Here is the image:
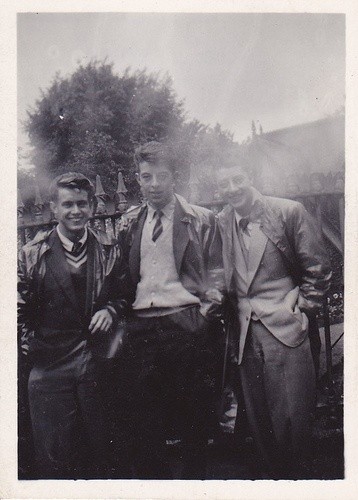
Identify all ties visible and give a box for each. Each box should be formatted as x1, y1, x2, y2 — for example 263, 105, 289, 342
152, 209, 163, 243
241, 218, 248, 238
71, 242, 82, 258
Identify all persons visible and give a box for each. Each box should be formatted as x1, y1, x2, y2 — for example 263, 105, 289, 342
213, 150, 336, 479
17, 170, 124, 480
114, 139, 229, 480
